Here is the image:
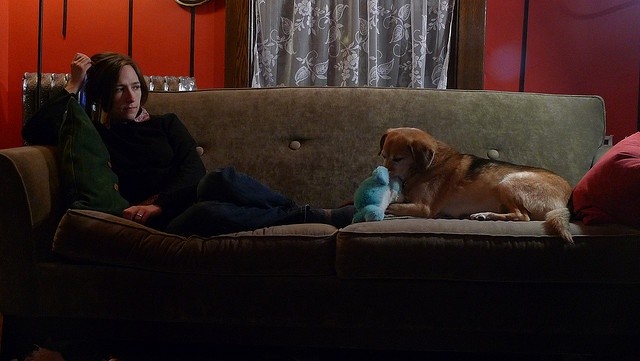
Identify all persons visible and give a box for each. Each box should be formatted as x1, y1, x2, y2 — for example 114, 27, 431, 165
17, 52, 356, 235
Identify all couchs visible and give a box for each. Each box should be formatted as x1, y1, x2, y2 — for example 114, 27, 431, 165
0, 85, 640, 331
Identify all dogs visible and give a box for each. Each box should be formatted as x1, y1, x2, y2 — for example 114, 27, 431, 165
378, 127, 576, 247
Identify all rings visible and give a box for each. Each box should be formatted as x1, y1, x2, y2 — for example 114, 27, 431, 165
136, 214, 143, 218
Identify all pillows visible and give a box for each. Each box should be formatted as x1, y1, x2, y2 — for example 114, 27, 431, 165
570, 128, 640, 228
54, 97, 129, 219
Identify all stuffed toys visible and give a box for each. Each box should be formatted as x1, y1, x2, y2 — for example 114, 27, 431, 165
348, 166, 405, 227
